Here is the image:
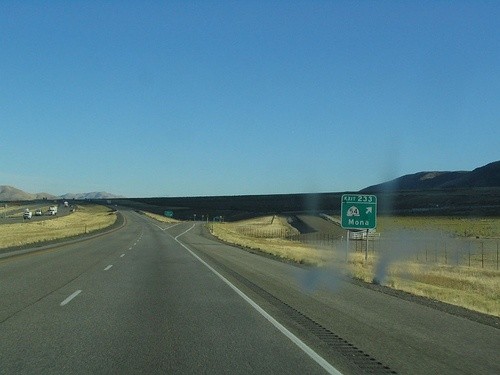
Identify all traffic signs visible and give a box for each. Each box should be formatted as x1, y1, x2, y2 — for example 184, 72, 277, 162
340, 194, 378, 231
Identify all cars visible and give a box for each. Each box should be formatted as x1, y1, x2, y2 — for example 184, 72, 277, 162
347, 228, 380, 242
24, 201, 73, 221
